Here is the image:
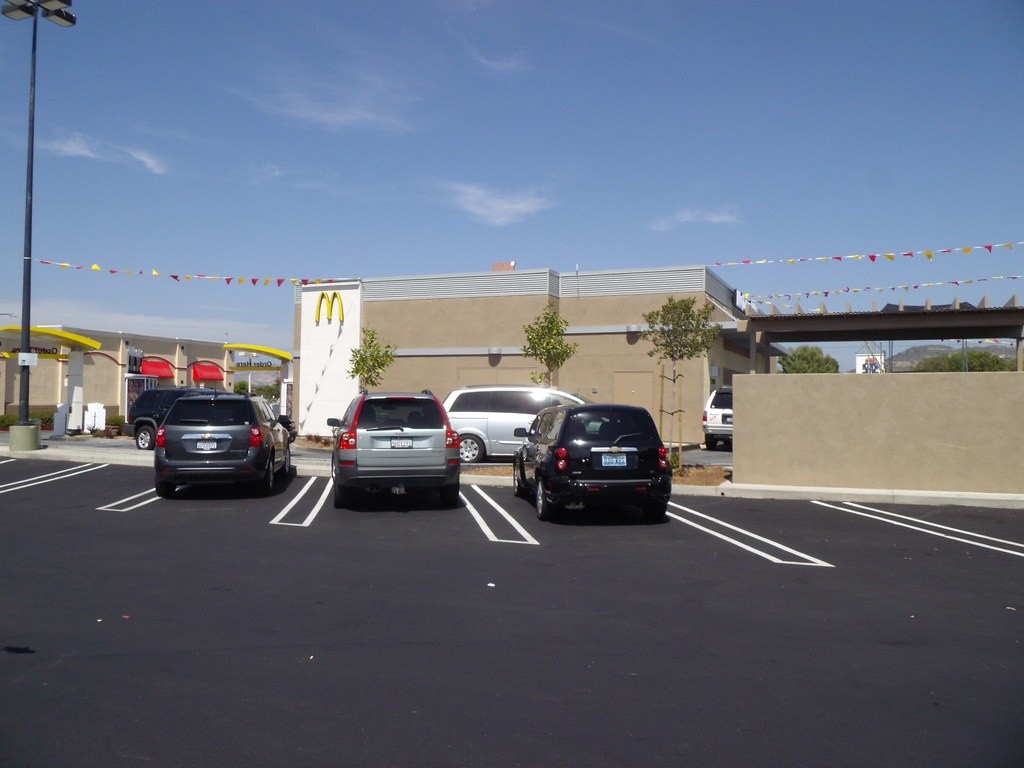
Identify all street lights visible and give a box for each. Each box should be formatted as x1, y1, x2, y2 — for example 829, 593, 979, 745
239, 351, 258, 395
1, 0, 78, 424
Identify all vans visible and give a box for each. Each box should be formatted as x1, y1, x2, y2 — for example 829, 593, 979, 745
444, 386, 610, 463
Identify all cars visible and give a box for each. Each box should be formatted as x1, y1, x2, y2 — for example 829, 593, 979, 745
270, 403, 280, 419
515, 402, 672, 523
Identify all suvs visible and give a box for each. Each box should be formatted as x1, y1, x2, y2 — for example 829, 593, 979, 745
126, 388, 298, 450
153, 395, 292, 496
701, 387, 734, 450
327, 387, 461, 507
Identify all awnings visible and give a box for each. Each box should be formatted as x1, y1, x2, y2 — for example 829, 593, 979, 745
193, 364, 224, 381
141, 361, 174, 379
